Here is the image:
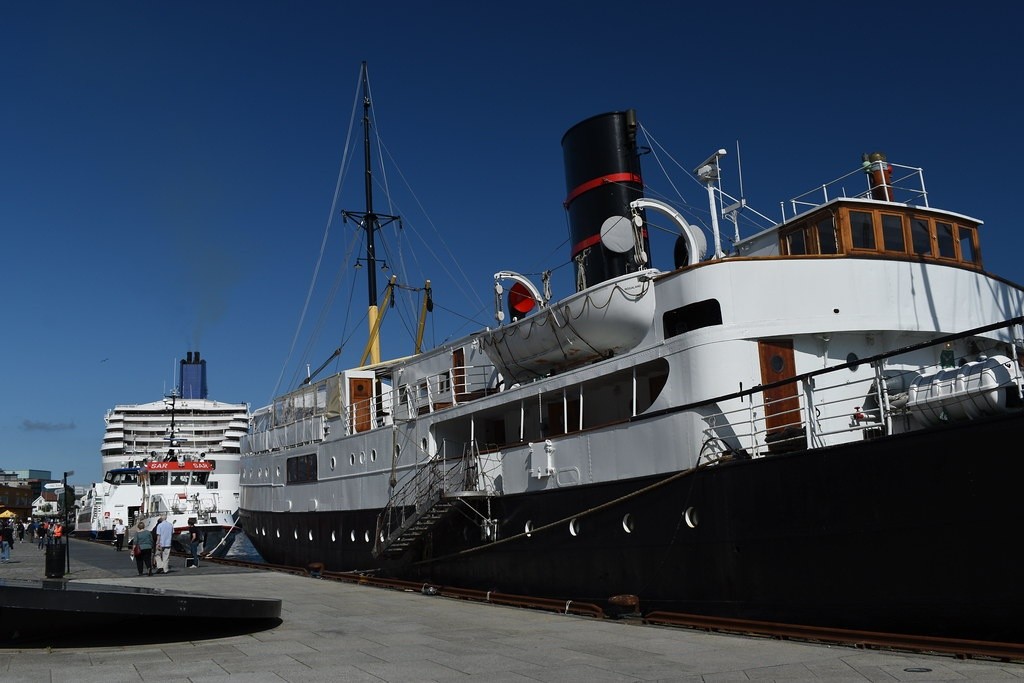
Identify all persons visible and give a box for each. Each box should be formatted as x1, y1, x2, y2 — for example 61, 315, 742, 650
130, 522, 156, 576
0, 519, 63, 564
188, 518, 202, 569
154, 515, 173, 574
114, 519, 126, 551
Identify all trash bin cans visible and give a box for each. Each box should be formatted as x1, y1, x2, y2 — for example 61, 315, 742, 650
45, 542, 67, 578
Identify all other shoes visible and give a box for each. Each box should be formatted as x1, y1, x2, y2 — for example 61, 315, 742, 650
147, 568, 153, 576
155, 568, 163, 574
137, 573, 143, 576
189, 565, 197, 568
163, 572, 167, 574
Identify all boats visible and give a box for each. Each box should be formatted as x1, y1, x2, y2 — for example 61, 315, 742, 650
68, 358, 252, 558
230, 62, 1023, 643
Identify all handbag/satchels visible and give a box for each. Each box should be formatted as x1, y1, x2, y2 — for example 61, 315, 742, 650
134, 544, 141, 555
